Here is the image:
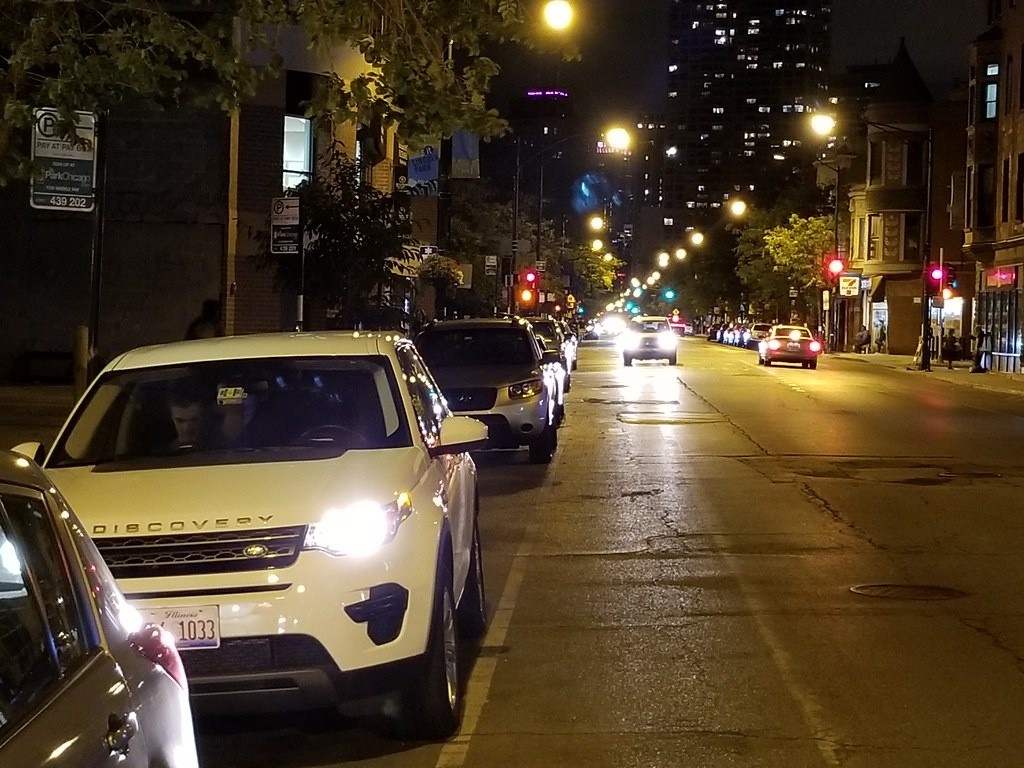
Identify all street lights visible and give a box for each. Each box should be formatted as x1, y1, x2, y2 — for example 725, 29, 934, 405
813, 115, 934, 371
511, 128, 629, 316
773, 148, 840, 354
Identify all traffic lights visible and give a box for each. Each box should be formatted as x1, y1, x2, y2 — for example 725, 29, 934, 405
931, 270, 942, 279
526, 273, 535, 282
521, 289, 532, 301
665, 292, 673, 299
947, 266, 957, 288
829, 260, 843, 273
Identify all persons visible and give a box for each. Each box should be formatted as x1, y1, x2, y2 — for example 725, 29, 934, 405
974, 324, 986, 367
115, 385, 243, 463
856, 325, 871, 353
946, 328, 959, 347
875, 319, 887, 354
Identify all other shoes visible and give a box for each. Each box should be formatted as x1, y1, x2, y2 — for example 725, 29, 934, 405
948, 367, 954, 370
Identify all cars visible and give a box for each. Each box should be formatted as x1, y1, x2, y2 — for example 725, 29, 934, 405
622, 316, 678, 366
758, 325, 820, 369
415, 315, 602, 466
669, 318, 685, 336
707, 323, 773, 349
685, 323, 694, 335
0, 448, 199, 767
11, 331, 488, 734
